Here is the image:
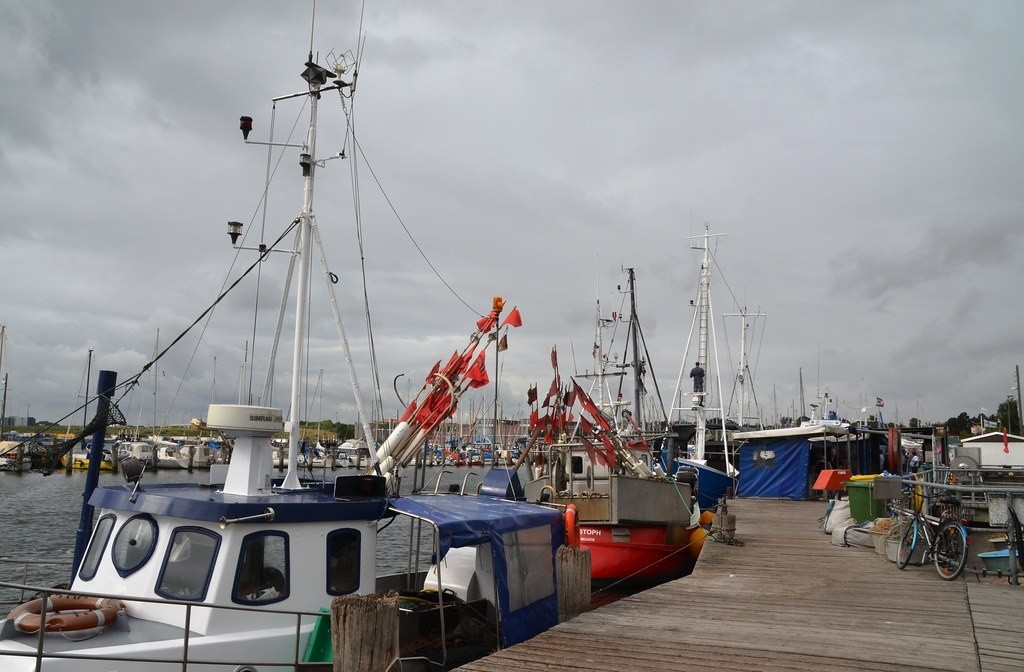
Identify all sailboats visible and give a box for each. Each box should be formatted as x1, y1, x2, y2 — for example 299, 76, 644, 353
0, 0, 567, 672
647, 225, 741, 516
1, 264, 918, 515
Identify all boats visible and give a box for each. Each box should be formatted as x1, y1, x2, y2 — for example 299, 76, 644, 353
471, 346, 713, 585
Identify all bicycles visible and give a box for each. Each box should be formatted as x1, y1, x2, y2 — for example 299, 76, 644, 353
894, 483, 971, 581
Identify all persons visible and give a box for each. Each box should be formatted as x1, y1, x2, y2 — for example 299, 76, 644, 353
901, 448, 920, 474
827, 446, 842, 501
689, 362, 706, 408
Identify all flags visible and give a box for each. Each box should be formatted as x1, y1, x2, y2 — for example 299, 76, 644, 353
876, 398, 883, 406
984, 420, 997, 428
971, 421, 986, 434
499, 334, 507, 353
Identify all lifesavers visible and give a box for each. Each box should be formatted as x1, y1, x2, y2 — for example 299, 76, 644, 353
8, 595, 126, 632
565, 503, 581, 549
